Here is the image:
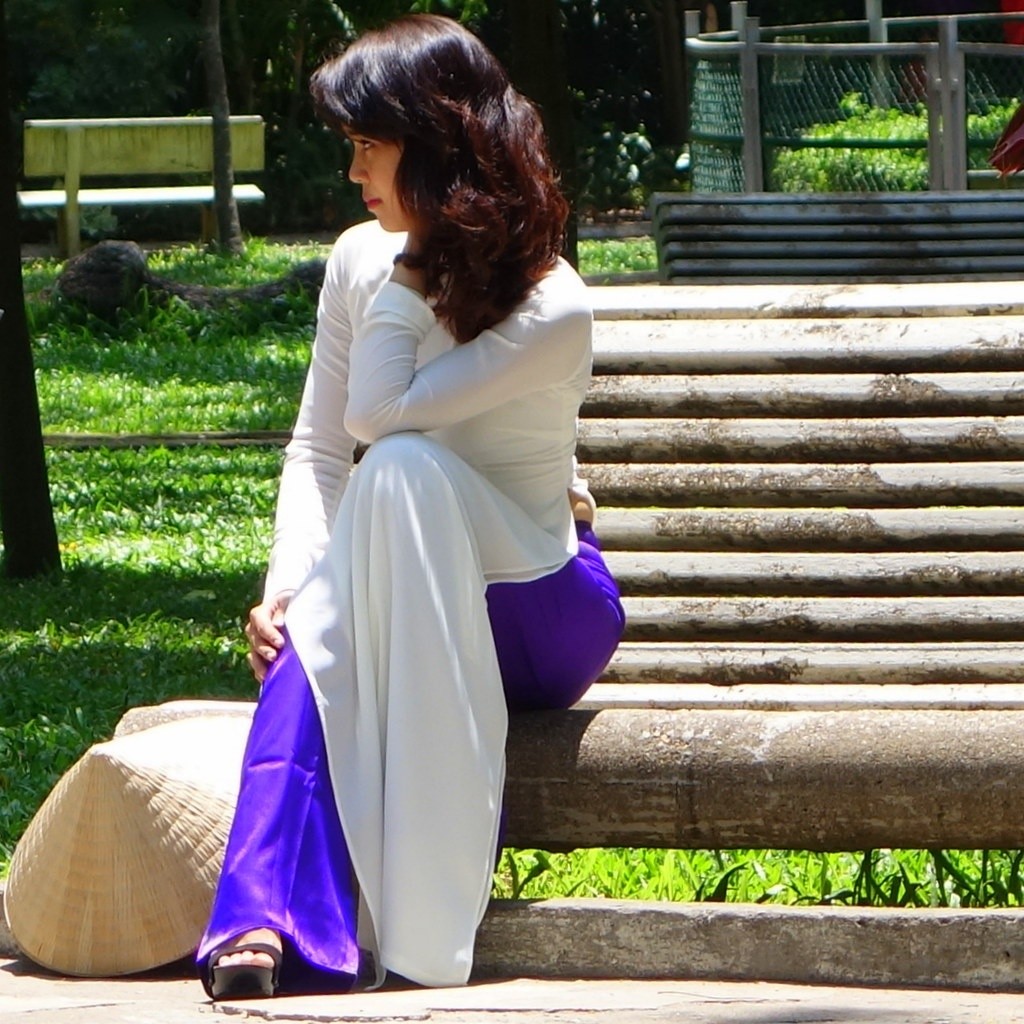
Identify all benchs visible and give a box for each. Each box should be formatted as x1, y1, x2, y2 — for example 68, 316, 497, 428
16, 115, 266, 254
648, 190, 1024, 288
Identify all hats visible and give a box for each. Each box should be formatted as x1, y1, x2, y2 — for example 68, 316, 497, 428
2, 716, 254, 977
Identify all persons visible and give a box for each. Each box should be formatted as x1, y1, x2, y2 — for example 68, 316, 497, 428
196, 13, 626, 1001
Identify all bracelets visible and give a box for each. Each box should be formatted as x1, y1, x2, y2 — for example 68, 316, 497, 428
393, 254, 430, 275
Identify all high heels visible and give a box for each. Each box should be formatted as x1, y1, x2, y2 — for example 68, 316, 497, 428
209, 929, 284, 996
